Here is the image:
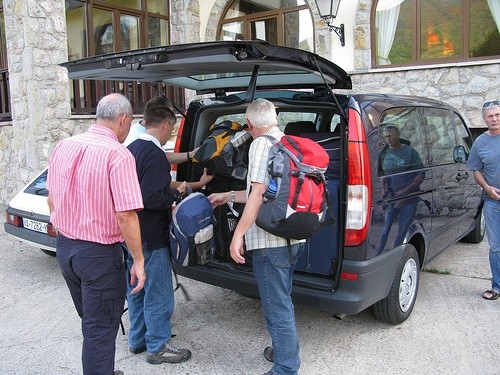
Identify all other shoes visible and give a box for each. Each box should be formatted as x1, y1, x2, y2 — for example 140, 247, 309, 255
262, 371, 274, 375
114, 370, 124, 375
264, 347, 275, 362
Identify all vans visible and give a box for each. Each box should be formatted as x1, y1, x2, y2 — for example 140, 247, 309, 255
59, 37, 486, 327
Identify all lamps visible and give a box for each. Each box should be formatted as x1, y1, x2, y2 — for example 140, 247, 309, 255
306, 0, 344, 45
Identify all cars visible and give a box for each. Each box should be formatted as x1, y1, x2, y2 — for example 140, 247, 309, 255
3, 140, 175, 258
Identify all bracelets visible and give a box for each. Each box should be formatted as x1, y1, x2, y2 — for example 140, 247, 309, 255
230, 191, 235, 202
186, 152, 190, 160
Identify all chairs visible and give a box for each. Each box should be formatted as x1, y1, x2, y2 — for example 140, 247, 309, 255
283, 121, 317, 137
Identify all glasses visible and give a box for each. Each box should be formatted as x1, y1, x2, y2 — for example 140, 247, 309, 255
483, 101, 500, 107
113, 242, 128, 273
383, 126, 394, 130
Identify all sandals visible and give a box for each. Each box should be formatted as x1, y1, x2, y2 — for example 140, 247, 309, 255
483, 290, 500, 300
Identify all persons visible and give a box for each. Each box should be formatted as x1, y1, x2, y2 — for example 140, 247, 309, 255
373, 124, 424, 259
202, 99, 307, 375
468, 101, 500, 300
120, 98, 214, 364
46, 93, 146, 375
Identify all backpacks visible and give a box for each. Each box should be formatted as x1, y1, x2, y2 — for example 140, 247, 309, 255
192, 120, 254, 192
168, 185, 215, 267
248, 135, 330, 240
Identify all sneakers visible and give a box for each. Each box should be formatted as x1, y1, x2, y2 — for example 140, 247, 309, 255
147, 341, 192, 364
130, 345, 147, 354
